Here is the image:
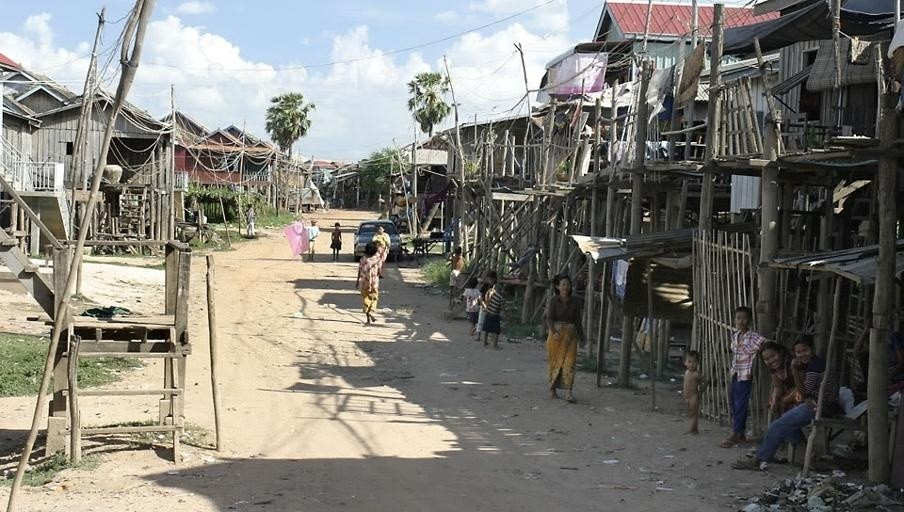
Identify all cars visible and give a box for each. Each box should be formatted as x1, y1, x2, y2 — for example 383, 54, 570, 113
354, 219, 403, 262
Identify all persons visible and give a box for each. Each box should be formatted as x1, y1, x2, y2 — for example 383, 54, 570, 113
355, 243, 382, 321
329, 222, 342, 260
307, 219, 319, 259
737, 334, 840, 470
446, 247, 464, 306
244, 204, 256, 236
720, 307, 766, 448
461, 271, 503, 350
371, 225, 391, 278
682, 351, 709, 435
546, 274, 587, 402
759, 342, 796, 427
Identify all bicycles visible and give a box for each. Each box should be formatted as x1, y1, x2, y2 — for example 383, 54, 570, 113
396, 238, 427, 268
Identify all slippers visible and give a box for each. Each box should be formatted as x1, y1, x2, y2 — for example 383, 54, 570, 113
565, 395, 577, 403
719, 439, 742, 448
